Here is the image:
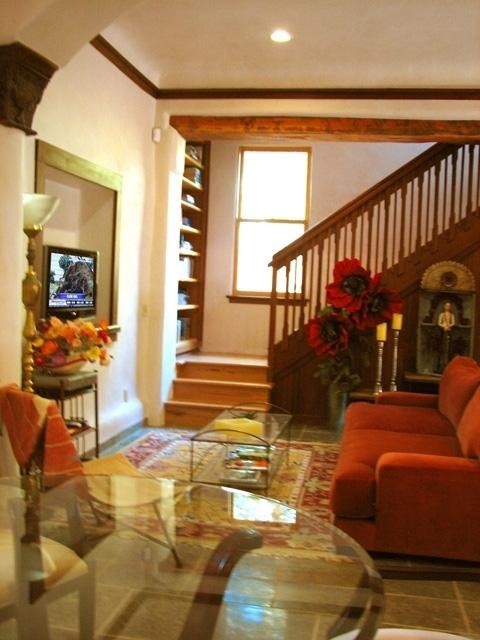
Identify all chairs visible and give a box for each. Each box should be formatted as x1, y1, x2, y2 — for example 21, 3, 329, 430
0, 380, 184, 570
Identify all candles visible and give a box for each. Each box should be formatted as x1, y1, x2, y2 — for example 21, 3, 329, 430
390, 312, 405, 331
375, 322, 388, 343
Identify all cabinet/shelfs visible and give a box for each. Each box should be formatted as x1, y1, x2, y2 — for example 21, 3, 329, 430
21, 367, 101, 464
175, 136, 211, 357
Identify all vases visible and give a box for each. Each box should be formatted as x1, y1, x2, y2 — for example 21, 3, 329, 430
36, 354, 90, 379
325, 378, 354, 433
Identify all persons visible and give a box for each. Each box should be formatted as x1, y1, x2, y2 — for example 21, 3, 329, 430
55, 255, 93, 297
433, 302, 456, 374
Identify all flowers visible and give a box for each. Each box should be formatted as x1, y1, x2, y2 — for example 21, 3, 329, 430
299, 254, 408, 391
29, 308, 115, 375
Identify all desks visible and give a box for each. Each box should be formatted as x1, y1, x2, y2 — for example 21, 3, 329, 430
347, 386, 402, 406
399, 369, 442, 394
0, 470, 388, 638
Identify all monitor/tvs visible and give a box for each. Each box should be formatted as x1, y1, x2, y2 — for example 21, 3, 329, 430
42, 245, 99, 317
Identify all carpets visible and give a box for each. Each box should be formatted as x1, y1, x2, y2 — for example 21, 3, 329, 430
106, 429, 344, 562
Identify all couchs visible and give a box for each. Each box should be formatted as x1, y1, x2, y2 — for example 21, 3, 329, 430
324, 352, 479, 579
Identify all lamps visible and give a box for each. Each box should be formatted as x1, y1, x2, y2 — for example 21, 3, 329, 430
18, 190, 59, 392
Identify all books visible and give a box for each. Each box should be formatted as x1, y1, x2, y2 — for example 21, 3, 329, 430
176, 142, 203, 343
220, 445, 277, 484
